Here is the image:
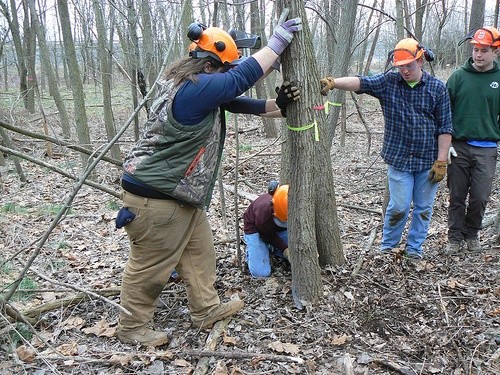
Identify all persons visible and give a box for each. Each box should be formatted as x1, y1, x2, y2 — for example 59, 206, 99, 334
320, 38, 453, 258
446, 26, 500, 254
117, 7, 303, 347
243, 184, 290, 278
168, 81, 300, 283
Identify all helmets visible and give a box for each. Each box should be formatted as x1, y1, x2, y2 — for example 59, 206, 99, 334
273, 185, 288, 222
392, 38, 424, 67
470, 26, 500, 46
188, 27, 239, 66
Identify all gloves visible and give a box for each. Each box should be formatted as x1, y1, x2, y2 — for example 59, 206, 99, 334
272, 56, 282, 72
267, 8, 302, 55
429, 160, 447, 184
275, 81, 300, 108
320, 77, 335, 96
447, 143, 458, 165
280, 107, 287, 118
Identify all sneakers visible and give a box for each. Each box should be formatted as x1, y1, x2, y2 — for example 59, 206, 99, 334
466, 239, 481, 253
118, 327, 171, 347
404, 253, 424, 271
194, 300, 244, 328
445, 240, 464, 255
